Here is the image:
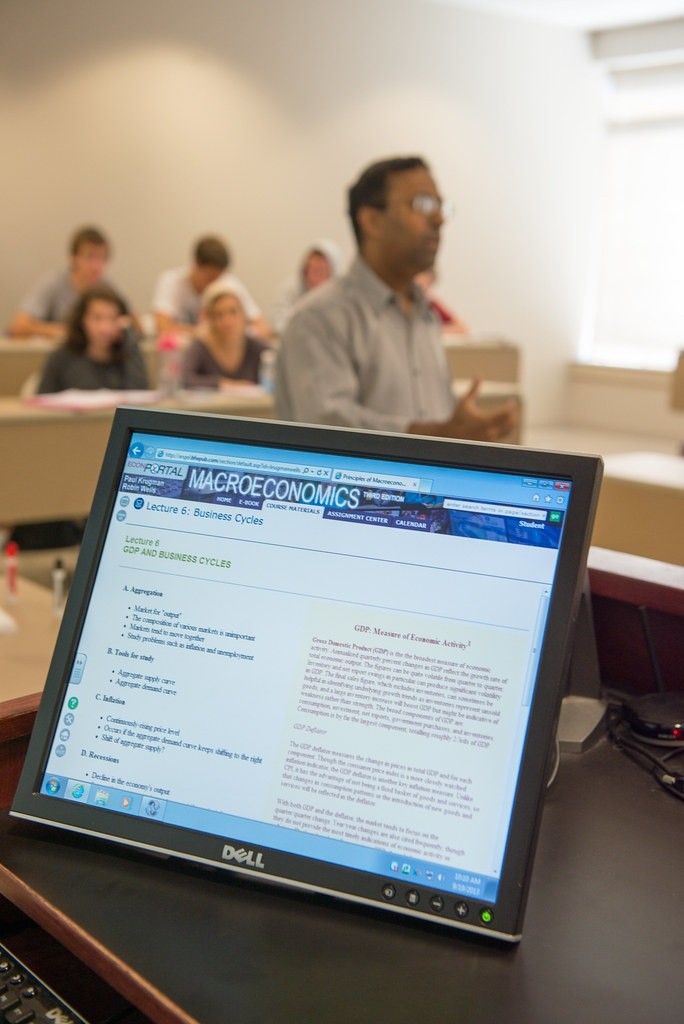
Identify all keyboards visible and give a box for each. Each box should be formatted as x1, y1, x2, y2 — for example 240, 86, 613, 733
0, 945, 88, 1024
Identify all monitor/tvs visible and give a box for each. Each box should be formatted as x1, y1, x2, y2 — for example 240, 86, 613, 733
7, 407, 604, 953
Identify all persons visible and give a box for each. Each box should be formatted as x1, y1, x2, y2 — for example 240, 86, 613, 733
413, 268, 462, 334
9, 226, 143, 339
37, 283, 147, 393
272, 158, 518, 442
144, 239, 271, 349
181, 277, 275, 393
300, 249, 332, 291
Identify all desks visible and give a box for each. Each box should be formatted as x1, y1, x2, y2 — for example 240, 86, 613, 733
0, 324, 683, 709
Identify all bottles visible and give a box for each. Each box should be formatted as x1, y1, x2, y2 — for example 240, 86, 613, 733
3, 541, 23, 604
155, 339, 183, 398
50, 556, 69, 617
258, 351, 277, 399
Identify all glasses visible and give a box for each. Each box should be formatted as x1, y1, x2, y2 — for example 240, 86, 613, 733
410, 196, 443, 216
